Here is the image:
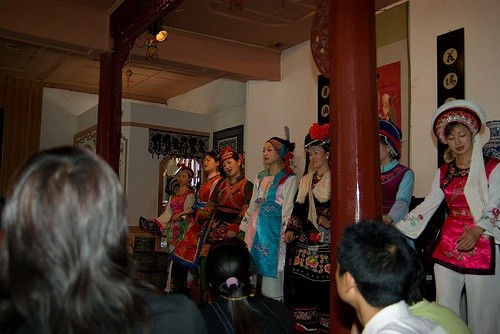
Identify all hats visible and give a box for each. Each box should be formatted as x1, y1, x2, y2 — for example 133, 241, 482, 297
378, 118, 403, 159
430, 100, 486, 145
174, 163, 194, 178
203, 148, 221, 162
219, 148, 246, 168
304, 123, 331, 152
266, 136, 295, 185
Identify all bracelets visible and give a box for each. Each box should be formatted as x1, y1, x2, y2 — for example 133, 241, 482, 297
184, 210, 187, 214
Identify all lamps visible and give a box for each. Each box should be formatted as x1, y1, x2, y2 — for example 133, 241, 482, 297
147, 17, 167, 42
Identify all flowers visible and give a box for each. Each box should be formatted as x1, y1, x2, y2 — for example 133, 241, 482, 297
308, 122, 330, 142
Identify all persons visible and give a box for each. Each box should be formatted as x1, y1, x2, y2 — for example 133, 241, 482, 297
238, 125, 298, 303
150, 146, 254, 292
0, 146, 208, 334
202, 238, 295, 334
395, 98, 500, 334
284, 122, 332, 334
336, 217, 472, 334
378, 119, 414, 253
